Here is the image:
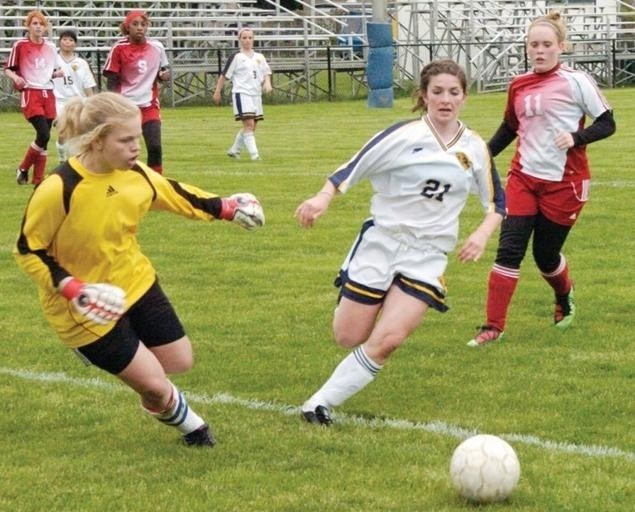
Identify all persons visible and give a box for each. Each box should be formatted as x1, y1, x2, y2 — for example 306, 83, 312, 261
294, 60, 509, 429
11, 92, 266, 449
3, 10, 65, 186
102, 11, 170, 176
53, 30, 97, 166
466, 11, 617, 348
212, 28, 273, 162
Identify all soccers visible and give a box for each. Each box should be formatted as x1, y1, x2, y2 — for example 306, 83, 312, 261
449, 434, 521, 503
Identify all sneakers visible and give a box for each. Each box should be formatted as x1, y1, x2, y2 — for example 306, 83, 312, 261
299, 406, 333, 425
182, 424, 214, 448
467, 324, 504, 347
251, 154, 262, 160
553, 281, 576, 330
228, 148, 241, 159
16, 167, 28, 185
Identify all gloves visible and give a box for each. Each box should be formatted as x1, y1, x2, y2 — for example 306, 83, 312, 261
219, 193, 266, 232
61, 277, 125, 325
